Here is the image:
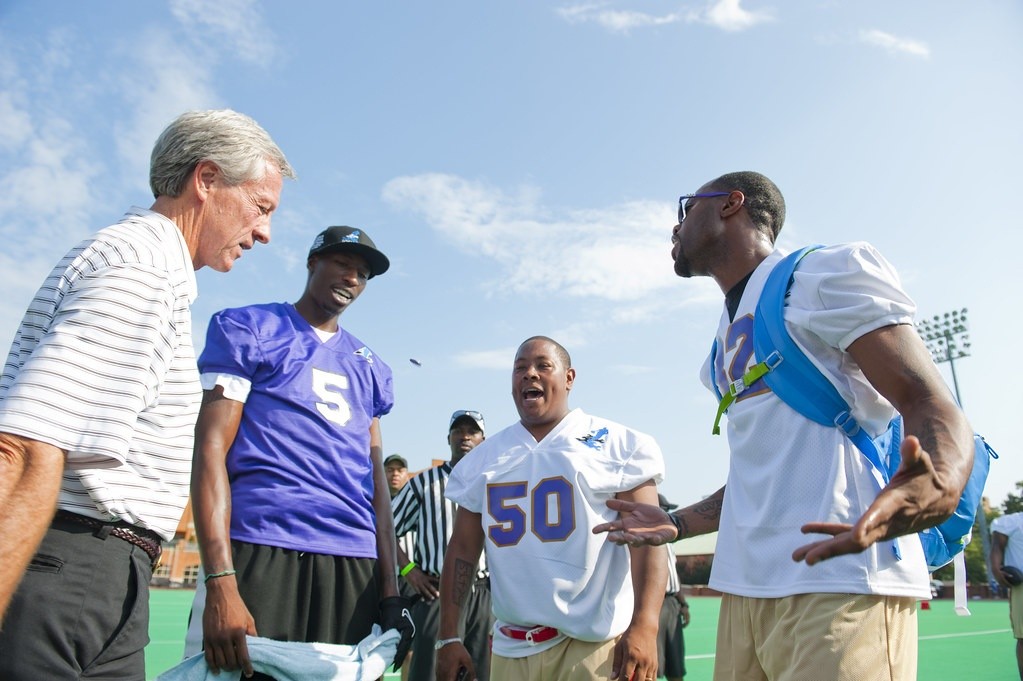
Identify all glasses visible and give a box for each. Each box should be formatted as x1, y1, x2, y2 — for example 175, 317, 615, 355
677, 193, 746, 226
451, 413, 484, 425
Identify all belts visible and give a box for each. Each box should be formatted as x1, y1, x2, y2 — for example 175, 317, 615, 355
54, 508, 161, 560
499, 623, 564, 646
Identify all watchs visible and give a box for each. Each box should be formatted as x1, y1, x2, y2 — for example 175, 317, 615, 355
435, 638, 461, 650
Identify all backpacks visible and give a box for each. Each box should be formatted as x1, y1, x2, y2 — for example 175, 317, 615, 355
711, 246, 998, 574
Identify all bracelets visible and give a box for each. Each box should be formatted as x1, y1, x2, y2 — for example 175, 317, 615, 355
401, 563, 416, 577
205, 570, 236, 583
681, 602, 688, 607
667, 512, 682, 542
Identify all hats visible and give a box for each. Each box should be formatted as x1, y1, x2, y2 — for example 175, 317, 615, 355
384, 455, 407, 468
448, 410, 485, 438
307, 226, 390, 279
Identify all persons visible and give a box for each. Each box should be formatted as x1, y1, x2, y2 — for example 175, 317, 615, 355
389, 410, 492, 681
0, 110, 295, 681
384, 455, 417, 681
990, 510, 1023, 681
658, 494, 690, 681
434, 336, 670, 681
183, 227, 415, 681
593, 170, 975, 681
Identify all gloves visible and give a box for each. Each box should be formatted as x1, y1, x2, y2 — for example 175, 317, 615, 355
377, 596, 416, 674
